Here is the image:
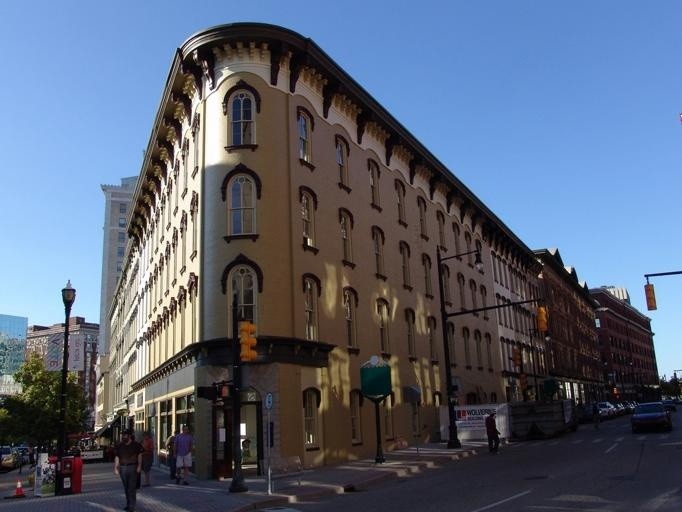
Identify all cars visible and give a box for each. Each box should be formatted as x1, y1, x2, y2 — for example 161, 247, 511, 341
597, 400, 639, 420
662, 399, 677, 413
630, 403, 673, 434
0, 446, 43, 472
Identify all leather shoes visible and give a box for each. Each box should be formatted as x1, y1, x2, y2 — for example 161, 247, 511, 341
123, 505, 136, 511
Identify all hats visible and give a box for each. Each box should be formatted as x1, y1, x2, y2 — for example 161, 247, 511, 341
120, 429, 135, 436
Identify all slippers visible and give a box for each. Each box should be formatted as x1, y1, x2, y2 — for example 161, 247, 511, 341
183, 480, 189, 485
175, 478, 181, 483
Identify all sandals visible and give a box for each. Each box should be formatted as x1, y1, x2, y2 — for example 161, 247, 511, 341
141, 483, 150, 486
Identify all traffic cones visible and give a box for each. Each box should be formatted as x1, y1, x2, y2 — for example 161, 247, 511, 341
14, 480, 24, 497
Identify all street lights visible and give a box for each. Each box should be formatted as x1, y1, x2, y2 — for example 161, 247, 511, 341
435, 240, 484, 450
54, 279, 76, 498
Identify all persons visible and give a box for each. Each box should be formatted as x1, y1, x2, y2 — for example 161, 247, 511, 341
173, 425, 194, 486
165, 430, 181, 481
140, 431, 154, 488
592, 401, 602, 431
484, 411, 503, 456
113, 428, 145, 512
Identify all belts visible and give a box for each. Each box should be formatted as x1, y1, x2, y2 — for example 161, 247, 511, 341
120, 462, 137, 466
